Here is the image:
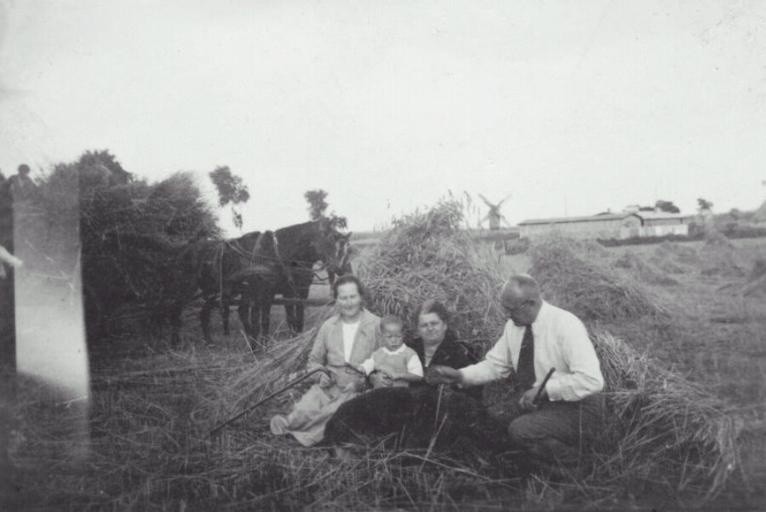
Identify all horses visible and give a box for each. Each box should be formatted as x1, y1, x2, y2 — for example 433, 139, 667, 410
168, 214, 356, 352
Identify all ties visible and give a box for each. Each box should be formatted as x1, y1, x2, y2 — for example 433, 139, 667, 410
517, 325, 536, 388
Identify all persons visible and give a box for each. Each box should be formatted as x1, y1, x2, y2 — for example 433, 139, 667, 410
270, 277, 382, 447
410, 300, 483, 401
425, 273, 604, 478
357, 317, 424, 389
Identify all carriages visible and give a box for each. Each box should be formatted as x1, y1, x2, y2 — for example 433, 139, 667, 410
0, 183, 353, 349
